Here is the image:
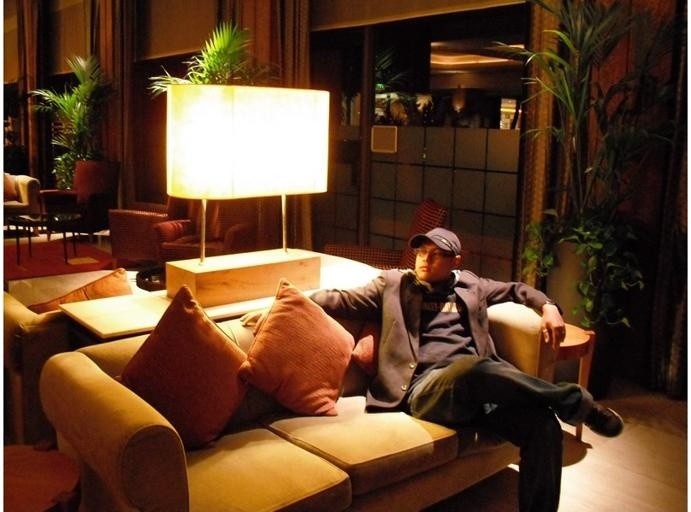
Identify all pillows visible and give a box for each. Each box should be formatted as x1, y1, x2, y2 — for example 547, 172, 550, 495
351, 315, 380, 378
117, 284, 255, 449
26, 264, 133, 315
234, 275, 357, 418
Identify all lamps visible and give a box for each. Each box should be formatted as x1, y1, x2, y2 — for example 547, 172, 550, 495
161, 82, 336, 310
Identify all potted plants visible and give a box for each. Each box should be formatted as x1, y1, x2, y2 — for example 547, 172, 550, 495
483, 0, 686, 400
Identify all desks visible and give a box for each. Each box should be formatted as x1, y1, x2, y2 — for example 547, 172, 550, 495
5, 439, 87, 511
554, 321, 598, 445
54, 264, 380, 353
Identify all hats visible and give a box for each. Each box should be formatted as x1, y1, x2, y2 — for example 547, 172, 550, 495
408, 226, 462, 258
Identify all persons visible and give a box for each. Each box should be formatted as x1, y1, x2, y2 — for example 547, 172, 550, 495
240, 228, 625, 512
4, 131, 26, 174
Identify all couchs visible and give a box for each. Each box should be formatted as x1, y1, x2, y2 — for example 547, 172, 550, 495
38, 273, 563, 511
3, 266, 139, 444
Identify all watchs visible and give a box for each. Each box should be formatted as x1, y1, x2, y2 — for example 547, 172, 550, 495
546, 299, 556, 305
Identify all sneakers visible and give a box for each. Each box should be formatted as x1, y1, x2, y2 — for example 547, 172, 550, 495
585, 403, 624, 438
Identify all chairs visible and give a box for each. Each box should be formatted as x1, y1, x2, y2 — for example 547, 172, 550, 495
321, 195, 462, 277
3, 151, 290, 270
